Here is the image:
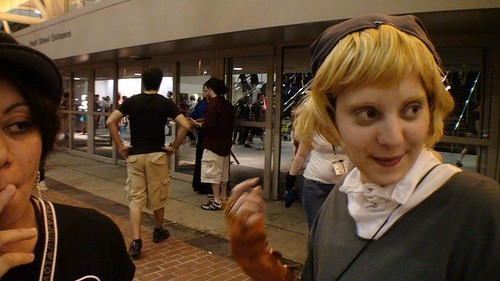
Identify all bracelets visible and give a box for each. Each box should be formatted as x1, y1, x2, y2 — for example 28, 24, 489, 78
283, 264, 300, 281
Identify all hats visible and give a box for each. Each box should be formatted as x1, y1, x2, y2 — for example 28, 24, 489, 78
0, 30, 63, 110
309, 12, 441, 74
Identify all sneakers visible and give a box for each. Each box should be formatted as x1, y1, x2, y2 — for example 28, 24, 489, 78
126, 240, 140, 256
153, 229, 170, 242
201, 201, 221, 210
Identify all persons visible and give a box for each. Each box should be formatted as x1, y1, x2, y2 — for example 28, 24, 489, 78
36, 76, 354, 234
456, 102, 482, 167
222, 13, 500, 281
107, 64, 193, 259
0, 30, 138, 281
